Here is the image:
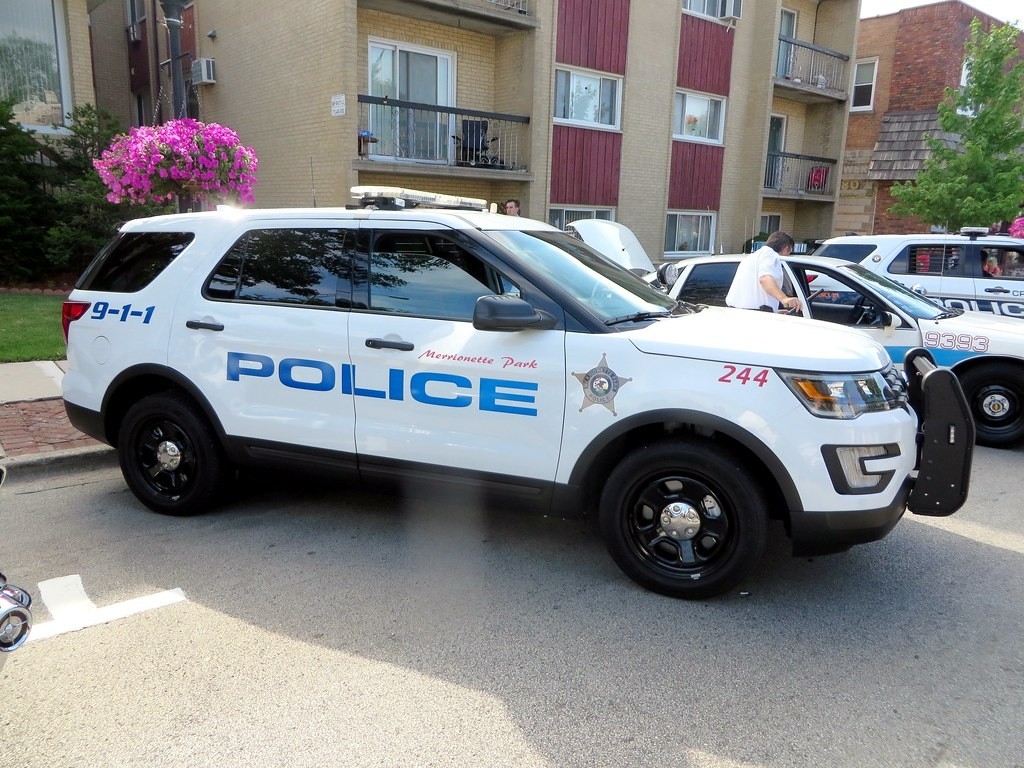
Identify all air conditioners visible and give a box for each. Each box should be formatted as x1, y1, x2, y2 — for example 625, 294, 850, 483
719, 0, 743, 20
191, 58, 213, 82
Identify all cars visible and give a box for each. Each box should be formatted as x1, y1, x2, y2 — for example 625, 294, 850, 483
61, 185, 919, 599
565, 219, 1024, 449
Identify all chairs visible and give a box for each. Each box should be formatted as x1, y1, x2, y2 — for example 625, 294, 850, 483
452, 120, 498, 162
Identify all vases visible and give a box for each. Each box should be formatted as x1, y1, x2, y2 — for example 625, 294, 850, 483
178, 178, 202, 192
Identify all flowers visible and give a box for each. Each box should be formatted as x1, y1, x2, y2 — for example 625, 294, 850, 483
92, 118, 258, 211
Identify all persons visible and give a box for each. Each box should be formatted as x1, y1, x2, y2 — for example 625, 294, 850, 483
725, 231, 803, 313
505, 199, 521, 217
982, 250, 1000, 278
